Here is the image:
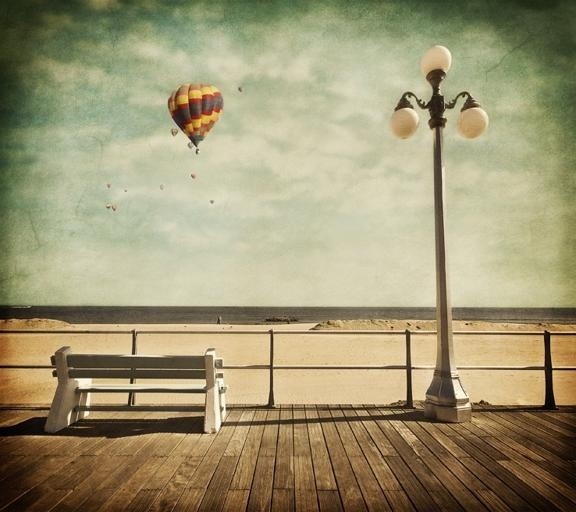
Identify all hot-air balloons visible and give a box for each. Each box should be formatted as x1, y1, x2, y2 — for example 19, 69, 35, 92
96, 82, 242, 215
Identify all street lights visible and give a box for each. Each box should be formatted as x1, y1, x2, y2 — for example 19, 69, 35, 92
384, 45, 493, 425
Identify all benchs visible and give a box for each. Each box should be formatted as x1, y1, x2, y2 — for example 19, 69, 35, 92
44, 346, 228, 434
